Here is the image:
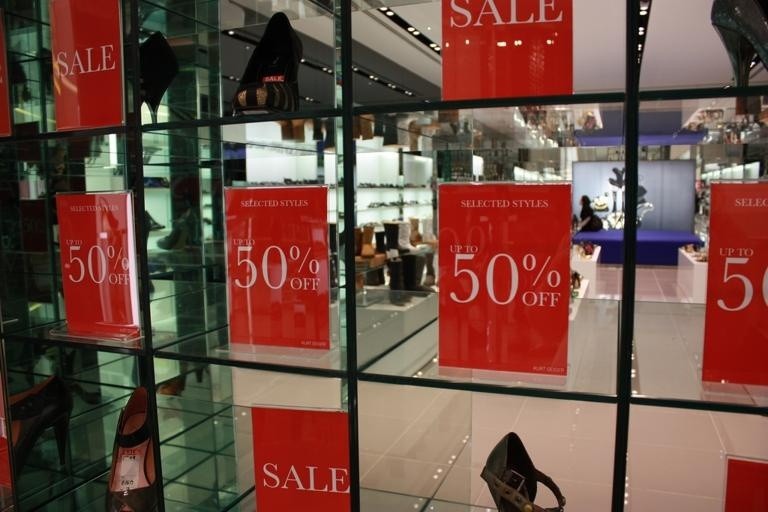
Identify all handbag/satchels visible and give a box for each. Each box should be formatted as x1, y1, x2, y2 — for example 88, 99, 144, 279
588, 215, 602, 231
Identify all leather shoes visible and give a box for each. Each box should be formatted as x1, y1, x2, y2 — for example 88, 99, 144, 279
480, 432, 566, 512
73, 384, 107, 406
105, 386, 160, 512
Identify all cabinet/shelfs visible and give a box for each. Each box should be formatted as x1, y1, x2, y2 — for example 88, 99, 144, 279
1, 1, 768, 512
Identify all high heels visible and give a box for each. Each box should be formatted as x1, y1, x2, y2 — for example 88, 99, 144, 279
157, 219, 194, 251
139, 30, 179, 124
155, 366, 204, 396
231, 12, 303, 117
710, 0, 768, 115
9, 374, 73, 481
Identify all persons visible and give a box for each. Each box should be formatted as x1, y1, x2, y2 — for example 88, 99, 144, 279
577, 194, 595, 260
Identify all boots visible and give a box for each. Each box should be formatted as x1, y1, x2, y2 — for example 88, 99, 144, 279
352, 113, 409, 145
276, 117, 335, 149
353, 219, 436, 306
